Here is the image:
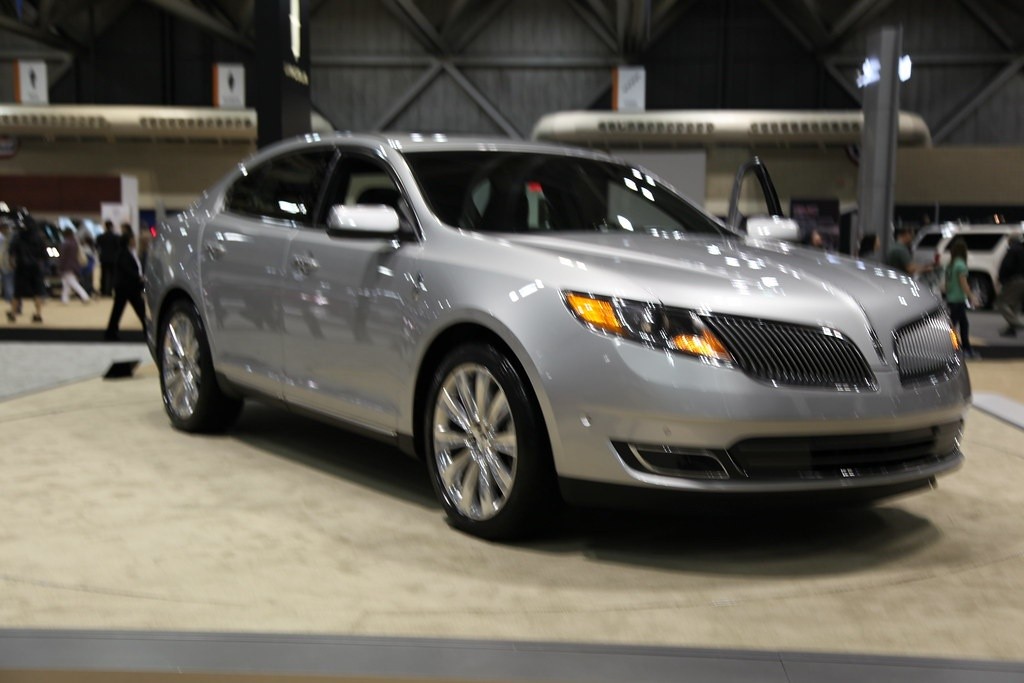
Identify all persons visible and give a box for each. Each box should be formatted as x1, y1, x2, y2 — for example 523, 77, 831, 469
945, 239, 981, 357
858, 227, 940, 296
207, 249, 374, 343
998, 233, 1024, 337
0, 218, 147, 334
792, 198, 823, 245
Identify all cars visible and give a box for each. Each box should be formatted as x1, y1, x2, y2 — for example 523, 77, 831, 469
137, 130, 977, 546
910, 221, 1024, 311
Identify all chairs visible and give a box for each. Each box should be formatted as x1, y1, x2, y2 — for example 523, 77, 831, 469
539, 168, 596, 232
355, 186, 403, 217
431, 171, 487, 231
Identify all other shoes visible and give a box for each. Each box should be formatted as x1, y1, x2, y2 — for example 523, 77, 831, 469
6, 311, 14, 321
33, 315, 42, 321
